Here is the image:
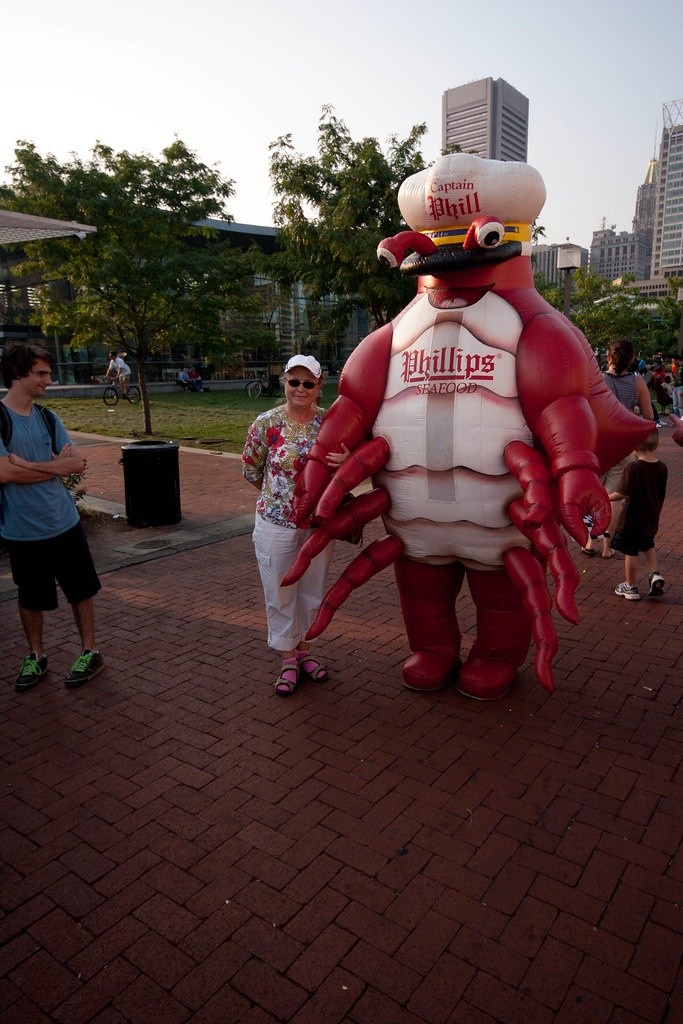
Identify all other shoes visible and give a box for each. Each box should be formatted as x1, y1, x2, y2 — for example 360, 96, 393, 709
122, 393, 128, 399
656, 423, 661, 427
659, 419, 668, 426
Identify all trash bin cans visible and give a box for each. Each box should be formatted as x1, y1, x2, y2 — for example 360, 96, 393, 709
121, 441, 182, 528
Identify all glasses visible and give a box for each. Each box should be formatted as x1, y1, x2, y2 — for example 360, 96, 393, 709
285, 378, 320, 389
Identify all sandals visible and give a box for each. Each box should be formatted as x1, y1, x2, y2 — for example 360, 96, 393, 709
295, 654, 328, 683
274, 665, 300, 696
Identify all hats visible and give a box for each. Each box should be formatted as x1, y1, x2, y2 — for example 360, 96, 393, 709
284, 354, 322, 379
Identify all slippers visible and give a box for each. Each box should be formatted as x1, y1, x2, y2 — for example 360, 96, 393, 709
602, 549, 615, 559
580, 546, 595, 554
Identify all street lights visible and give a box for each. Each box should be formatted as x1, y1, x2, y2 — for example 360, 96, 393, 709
554, 245, 583, 321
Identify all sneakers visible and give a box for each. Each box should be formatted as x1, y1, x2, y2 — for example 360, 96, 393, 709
615, 582, 641, 600
15, 651, 49, 689
64, 648, 106, 687
648, 571, 665, 597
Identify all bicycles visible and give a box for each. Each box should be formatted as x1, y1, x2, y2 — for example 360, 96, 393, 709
245, 370, 281, 399
102, 377, 141, 407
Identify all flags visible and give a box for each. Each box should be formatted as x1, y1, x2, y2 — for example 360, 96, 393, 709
571, 514, 611, 541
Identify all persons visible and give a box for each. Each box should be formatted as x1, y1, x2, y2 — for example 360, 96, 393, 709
242, 354, 351, 697
107, 351, 131, 395
582, 339, 654, 557
629, 351, 683, 448
0, 341, 106, 691
609, 429, 668, 599
189, 366, 203, 392
177, 367, 192, 392
